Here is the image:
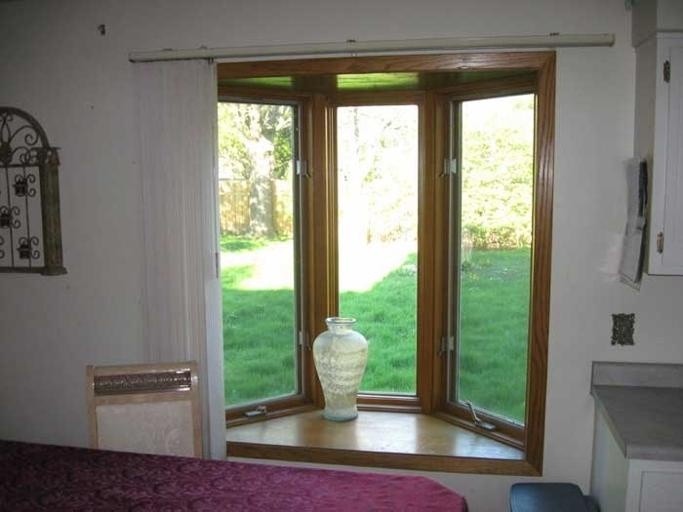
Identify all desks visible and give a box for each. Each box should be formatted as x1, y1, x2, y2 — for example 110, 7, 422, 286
1, 440, 469, 512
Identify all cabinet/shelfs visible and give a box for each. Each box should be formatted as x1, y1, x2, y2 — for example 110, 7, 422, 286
589, 402, 683, 512
630, 0, 683, 276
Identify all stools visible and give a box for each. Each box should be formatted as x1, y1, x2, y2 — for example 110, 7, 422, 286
509, 482, 590, 512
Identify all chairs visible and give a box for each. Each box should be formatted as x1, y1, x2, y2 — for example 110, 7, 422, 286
85, 361, 203, 459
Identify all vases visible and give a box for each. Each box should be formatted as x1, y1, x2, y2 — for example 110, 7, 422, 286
312, 317, 368, 422
